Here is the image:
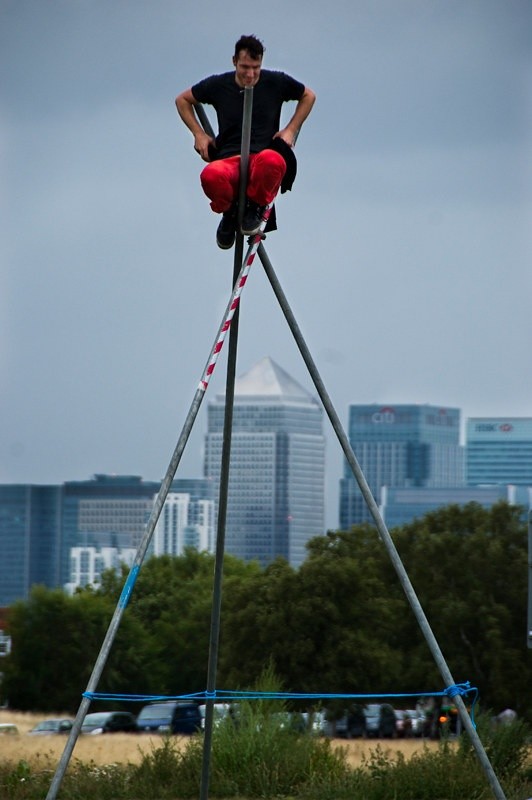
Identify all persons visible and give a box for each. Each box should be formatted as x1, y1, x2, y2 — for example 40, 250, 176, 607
174, 35, 316, 249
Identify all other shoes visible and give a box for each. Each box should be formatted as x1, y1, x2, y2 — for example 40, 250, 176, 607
216, 215, 235, 248
241, 201, 265, 235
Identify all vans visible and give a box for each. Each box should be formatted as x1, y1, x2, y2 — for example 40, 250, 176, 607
134, 701, 241, 736
79, 712, 139, 735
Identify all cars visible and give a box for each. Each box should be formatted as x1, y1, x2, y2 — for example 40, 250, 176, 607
27, 719, 72, 734
268, 702, 466, 738
